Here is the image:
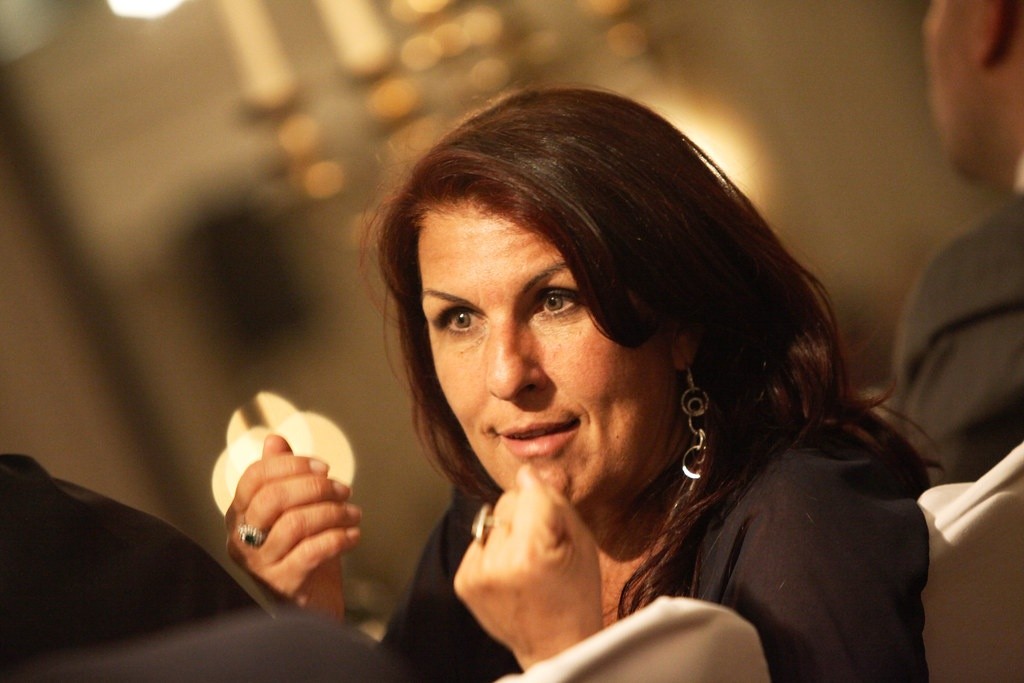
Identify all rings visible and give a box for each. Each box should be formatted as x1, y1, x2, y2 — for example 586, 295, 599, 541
238, 513, 265, 547
471, 500, 507, 545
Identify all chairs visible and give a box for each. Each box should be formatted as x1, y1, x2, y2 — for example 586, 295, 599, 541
495, 597, 773, 683
914, 435, 1023, 682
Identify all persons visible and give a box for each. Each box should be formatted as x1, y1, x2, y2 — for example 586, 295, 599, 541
0, 447, 268, 683
226, 86, 930, 683
895, 0, 1024, 488
